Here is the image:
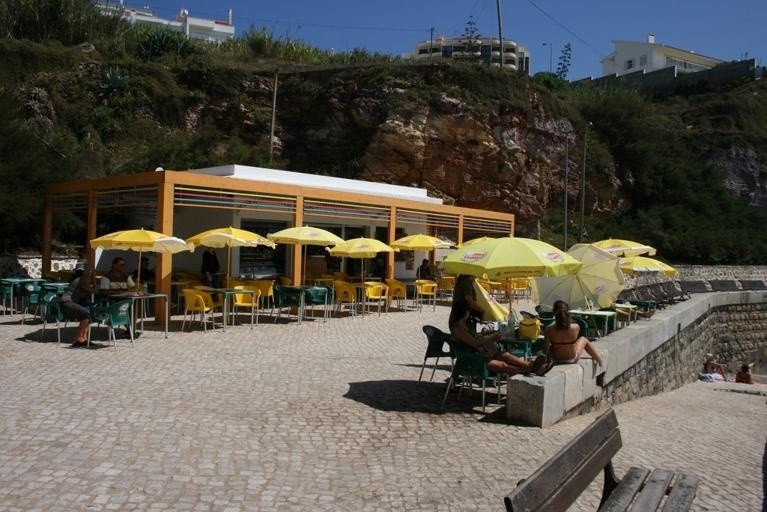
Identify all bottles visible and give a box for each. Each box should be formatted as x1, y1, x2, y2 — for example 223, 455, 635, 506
488, 311, 515, 337
135, 283, 148, 292
587, 300, 594, 312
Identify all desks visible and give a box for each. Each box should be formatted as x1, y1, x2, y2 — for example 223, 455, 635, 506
541, 299, 660, 336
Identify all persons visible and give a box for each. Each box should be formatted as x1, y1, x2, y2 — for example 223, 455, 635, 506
704, 354, 727, 379
446, 296, 554, 378
100, 256, 151, 339
735, 360, 756, 384
443, 274, 485, 386
200, 245, 219, 314
58, 261, 97, 346
543, 300, 604, 367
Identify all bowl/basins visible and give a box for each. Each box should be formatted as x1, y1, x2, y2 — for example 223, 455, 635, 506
240, 273, 254, 279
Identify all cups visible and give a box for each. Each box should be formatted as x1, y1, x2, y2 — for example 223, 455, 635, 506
625, 301, 630, 305
128, 291, 142, 295
110, 282, 127, 287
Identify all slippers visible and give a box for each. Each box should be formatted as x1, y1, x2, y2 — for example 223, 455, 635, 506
532, 353, 554, 376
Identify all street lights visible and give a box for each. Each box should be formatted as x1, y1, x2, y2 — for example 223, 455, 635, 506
556, 122, 572, 252
541, 42, 552, 73
266, 66, 280, 166
579, 119, 592, 244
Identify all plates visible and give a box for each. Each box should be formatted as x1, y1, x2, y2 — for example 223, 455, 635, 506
110, 287, 127, 289
129, 295, 144, 297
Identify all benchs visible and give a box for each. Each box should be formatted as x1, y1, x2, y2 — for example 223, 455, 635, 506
502, 407, 701, 512
619, 279, 767, 312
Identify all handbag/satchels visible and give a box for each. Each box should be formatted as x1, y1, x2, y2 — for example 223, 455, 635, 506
517, 317, 541, 340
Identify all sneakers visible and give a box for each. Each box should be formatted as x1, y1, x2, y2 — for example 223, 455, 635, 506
70, 339, 97, 348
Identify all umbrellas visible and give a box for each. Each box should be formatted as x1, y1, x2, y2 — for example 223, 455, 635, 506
441, 231, 582, 315
612, 253, 679, 297
589, 237, 659, 257
528, 241, 627, 337
